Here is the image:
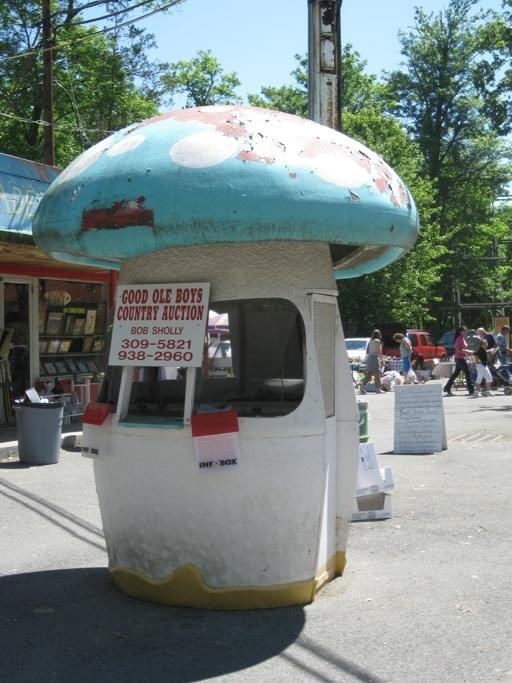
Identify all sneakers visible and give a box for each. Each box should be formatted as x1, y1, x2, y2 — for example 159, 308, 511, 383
444, 386, 497, 398
359, 386, 384, 394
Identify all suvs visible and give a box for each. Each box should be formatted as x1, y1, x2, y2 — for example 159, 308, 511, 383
379, 321, 447, 361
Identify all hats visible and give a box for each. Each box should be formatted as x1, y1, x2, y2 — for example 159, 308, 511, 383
477, 327, 486, 334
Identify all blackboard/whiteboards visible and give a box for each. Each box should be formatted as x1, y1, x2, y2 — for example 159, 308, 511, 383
394, 384, 442, 453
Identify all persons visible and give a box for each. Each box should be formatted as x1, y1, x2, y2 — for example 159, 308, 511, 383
358, 328, 387, 394
393, 332, 412, 383
444, 324, 511, 397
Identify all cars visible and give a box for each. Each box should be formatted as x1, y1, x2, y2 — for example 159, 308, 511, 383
345, 336, 371, 368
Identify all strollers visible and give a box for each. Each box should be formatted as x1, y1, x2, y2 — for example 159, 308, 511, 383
467, 344, 512, 395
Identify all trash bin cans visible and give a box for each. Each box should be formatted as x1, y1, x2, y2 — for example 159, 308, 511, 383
12, 399, 64, 465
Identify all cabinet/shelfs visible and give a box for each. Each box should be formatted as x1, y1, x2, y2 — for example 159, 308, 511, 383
38, 298, 107, 376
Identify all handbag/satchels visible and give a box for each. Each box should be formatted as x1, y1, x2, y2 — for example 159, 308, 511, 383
362, 355, 369, 365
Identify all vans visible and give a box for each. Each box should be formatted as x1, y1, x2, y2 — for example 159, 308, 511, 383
440, 330, 456, 356
